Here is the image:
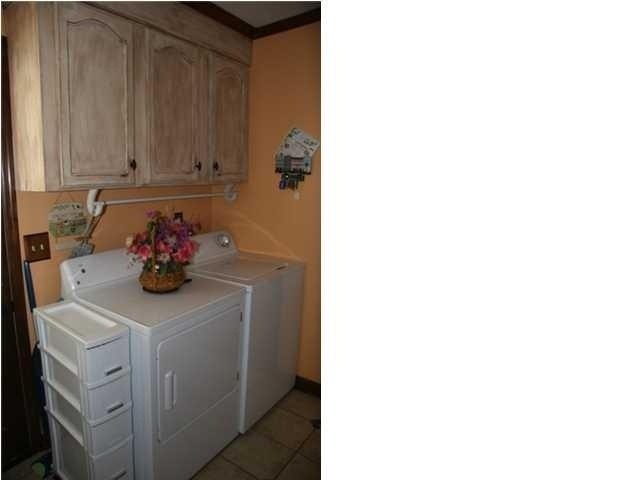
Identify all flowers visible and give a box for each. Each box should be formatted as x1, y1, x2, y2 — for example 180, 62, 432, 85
125, 212, 201, 272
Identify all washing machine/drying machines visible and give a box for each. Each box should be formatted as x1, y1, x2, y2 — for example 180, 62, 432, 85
60, 246, 248, 480
185, 231, 305, 435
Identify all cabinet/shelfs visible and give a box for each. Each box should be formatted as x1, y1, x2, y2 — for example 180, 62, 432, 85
34, 300, 133, 480
138, 1, 252, 187
4, 2, 138, 193
